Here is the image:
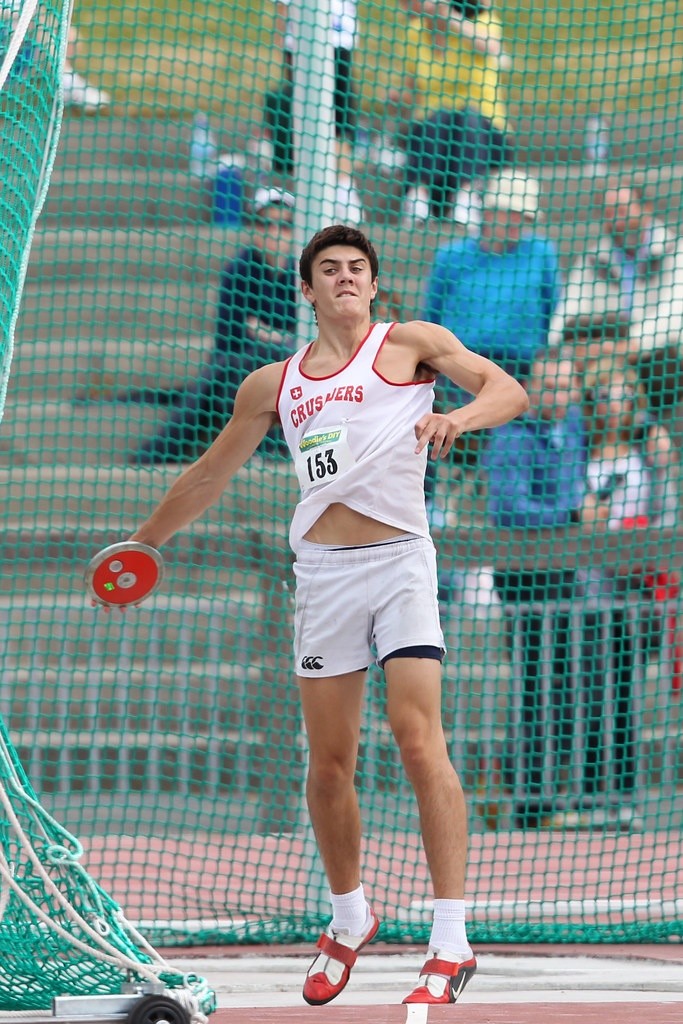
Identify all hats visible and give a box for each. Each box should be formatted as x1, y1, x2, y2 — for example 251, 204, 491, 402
248, 184, 296, 216
483, 167, 538, 220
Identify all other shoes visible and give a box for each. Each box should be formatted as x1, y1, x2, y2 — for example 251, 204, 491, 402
58, 74, 112, 113
510, 803, 642, 833
447, 188, 480, 234
401, 190, 431, 231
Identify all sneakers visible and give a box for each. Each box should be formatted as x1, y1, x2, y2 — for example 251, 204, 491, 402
302, 900, 380, 1006
401, 942, 477, 1003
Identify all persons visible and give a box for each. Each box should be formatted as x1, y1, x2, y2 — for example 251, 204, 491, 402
383, 0, 515, 225
266, 0, 364, 225
91, 226, 529, 1004
217, 184, 299, 463
375, 171, 683, 828
0, 0, 105, 109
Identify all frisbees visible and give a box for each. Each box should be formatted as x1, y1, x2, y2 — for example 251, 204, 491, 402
83, 541, 166, 608
